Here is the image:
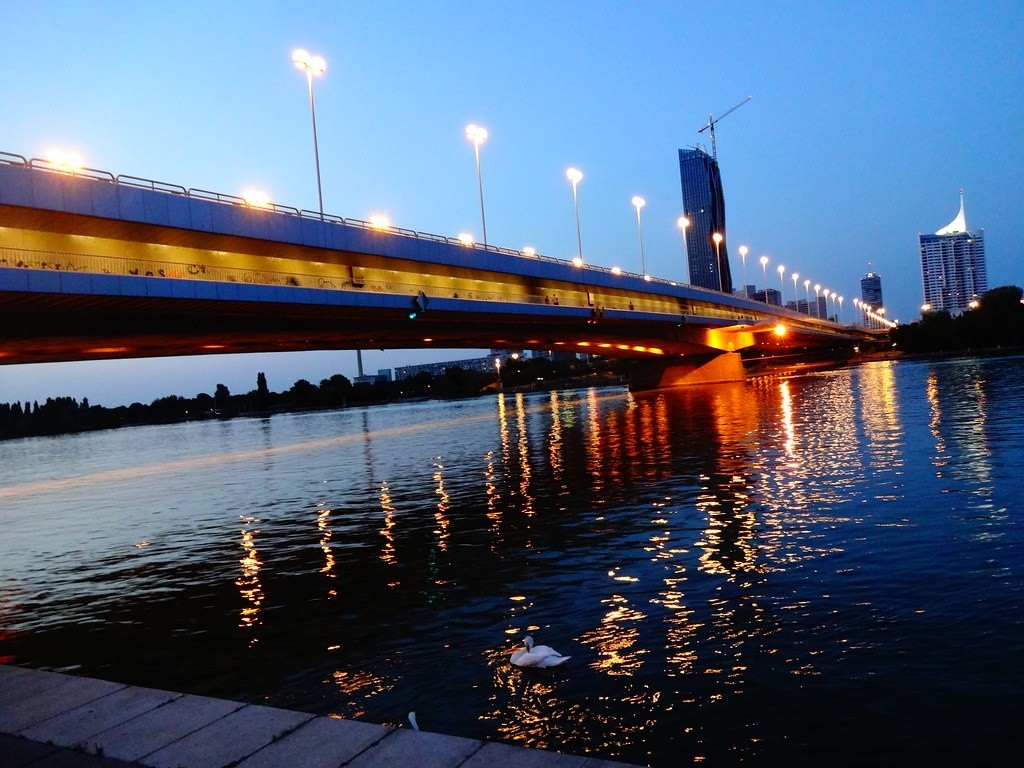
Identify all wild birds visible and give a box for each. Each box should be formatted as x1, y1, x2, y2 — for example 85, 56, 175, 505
509, 635, 572, 668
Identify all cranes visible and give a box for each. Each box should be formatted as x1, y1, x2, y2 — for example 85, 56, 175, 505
698, 95, 753, 160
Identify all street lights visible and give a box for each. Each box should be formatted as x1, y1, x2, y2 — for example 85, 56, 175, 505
462, 119, 489, 250
291, 47, 327, 223
564, 168, 899, 335
